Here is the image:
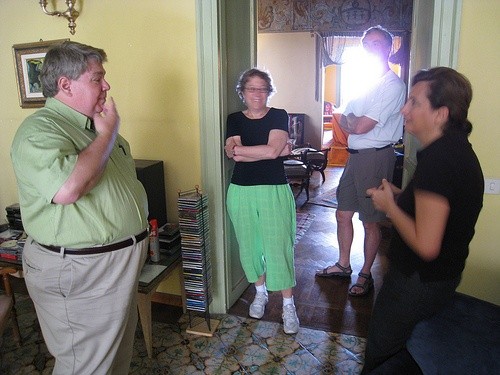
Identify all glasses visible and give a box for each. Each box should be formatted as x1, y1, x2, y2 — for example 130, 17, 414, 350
244, 87, 268, 93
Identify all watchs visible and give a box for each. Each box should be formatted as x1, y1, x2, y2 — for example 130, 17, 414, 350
231, 144, 237, 156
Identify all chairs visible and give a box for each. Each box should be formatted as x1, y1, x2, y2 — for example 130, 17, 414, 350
324, 102, 337, 130
0, 267, 24, 350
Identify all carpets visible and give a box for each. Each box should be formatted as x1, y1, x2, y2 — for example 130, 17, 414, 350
294, 209, 316, 250
306, 185, 359, 212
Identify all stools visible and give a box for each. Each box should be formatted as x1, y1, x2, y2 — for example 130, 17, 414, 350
405, 290, 500, 375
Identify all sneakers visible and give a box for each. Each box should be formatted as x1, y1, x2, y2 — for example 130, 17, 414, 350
282, 304, 299, 334
249, 291, 269, 319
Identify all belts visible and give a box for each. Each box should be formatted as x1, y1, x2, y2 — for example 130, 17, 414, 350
346, 143, 392, 154
37, 225, 149, 255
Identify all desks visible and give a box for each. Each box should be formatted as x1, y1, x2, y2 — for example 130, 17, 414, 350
0, 222, 186, 362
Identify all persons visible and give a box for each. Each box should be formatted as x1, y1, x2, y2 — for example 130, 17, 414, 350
223, 68, 300, 335
359, 68, 486, 374
314, 25, 409, 295
10, 41, 150, 374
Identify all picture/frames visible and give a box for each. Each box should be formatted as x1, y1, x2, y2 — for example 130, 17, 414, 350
12, 38, 70, 109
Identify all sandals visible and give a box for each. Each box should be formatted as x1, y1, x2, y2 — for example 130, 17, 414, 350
348, 272, 374, 296
316, 262, 353, 277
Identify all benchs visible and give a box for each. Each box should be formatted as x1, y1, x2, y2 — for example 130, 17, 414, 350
290, 142, 332, 186
281, 150, 310, 203
